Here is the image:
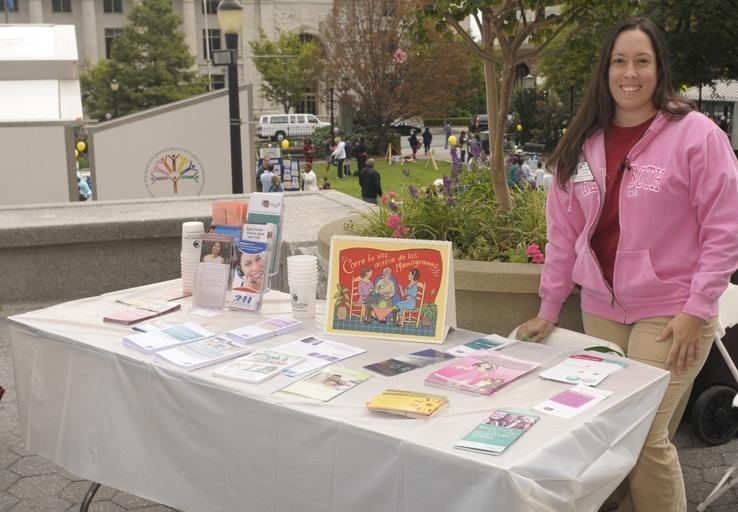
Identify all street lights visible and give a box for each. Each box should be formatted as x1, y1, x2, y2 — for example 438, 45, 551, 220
328, 79, 335, 146
110, 77, 120, 118
211, 0, 244, 192
569, 77, 576, 121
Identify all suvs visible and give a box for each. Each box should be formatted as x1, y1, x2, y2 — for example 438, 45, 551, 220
469, 115, 488, 132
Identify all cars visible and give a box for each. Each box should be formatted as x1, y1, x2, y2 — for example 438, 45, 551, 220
390, 120, 421, 136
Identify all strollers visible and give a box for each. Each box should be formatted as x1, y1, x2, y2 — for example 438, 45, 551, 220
686, 268, 738, 443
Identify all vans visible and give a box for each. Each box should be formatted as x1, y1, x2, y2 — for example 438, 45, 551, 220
256, 114, 332, 142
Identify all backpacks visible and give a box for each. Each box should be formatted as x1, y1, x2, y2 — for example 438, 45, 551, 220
418, 141, 423, 150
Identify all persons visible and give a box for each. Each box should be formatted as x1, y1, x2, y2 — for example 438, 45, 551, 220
322, 175, 329, 190
299, 162, 318, 193
359, 266, 375, 323
358, 157, 382, 205
511, 156, 521, 165
268, 175, 284, 194
259, 163, 276, 192
374, 267, 396, 325
520, 158, 531, 180
459, 131, 468, 163
410, 129, 421, 160
533, 162, 545, 190
201, 241, 225, 265
473, 114, 480, 131
445, 123, 452, 150
75, 176, 92, 200
467, 134, 477, 162
234, 245, 267, 291
395, 270, 419, 326
77, 185, 86, 202
355, 138, 369, 173
331, 137, 347, 181
303, 138, 314, 169
514, 18, 736, 512
256, 157, 270, 192
423, 126, 433, 155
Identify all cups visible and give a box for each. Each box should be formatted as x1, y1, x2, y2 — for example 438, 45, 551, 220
181, 222, 204, 293
287, 254, 318, 320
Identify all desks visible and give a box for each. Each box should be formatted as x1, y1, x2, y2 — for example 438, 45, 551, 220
4, 278, 671, 512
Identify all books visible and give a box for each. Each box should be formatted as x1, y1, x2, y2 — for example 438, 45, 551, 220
242, 224, 267, 241
536, 348, 627, 416
293, 177, 299, 181
274, 165, 281, 175
426, 350, 540, 398
155, 335, 251, 370
292, 182, 298, 188
268, 221, 278, 277
122, 324, 208, 352
366, 350, 456, 377
291, 161, 297, 170
285, 181, 291, 189
283, 175, 290, 179
455, 411, 540, 455
246, 193, 285, 223
451, 332, 515, 359
369, 388, 446, 419
284, 169, 291, 174
227, 313, 304, 344
291, 170, 298, 176
283, 160, 290, 167
283, 364, 373, 403
288, 335, 368, 362
212, 353, 307, 386
104, 301, 181, 325
